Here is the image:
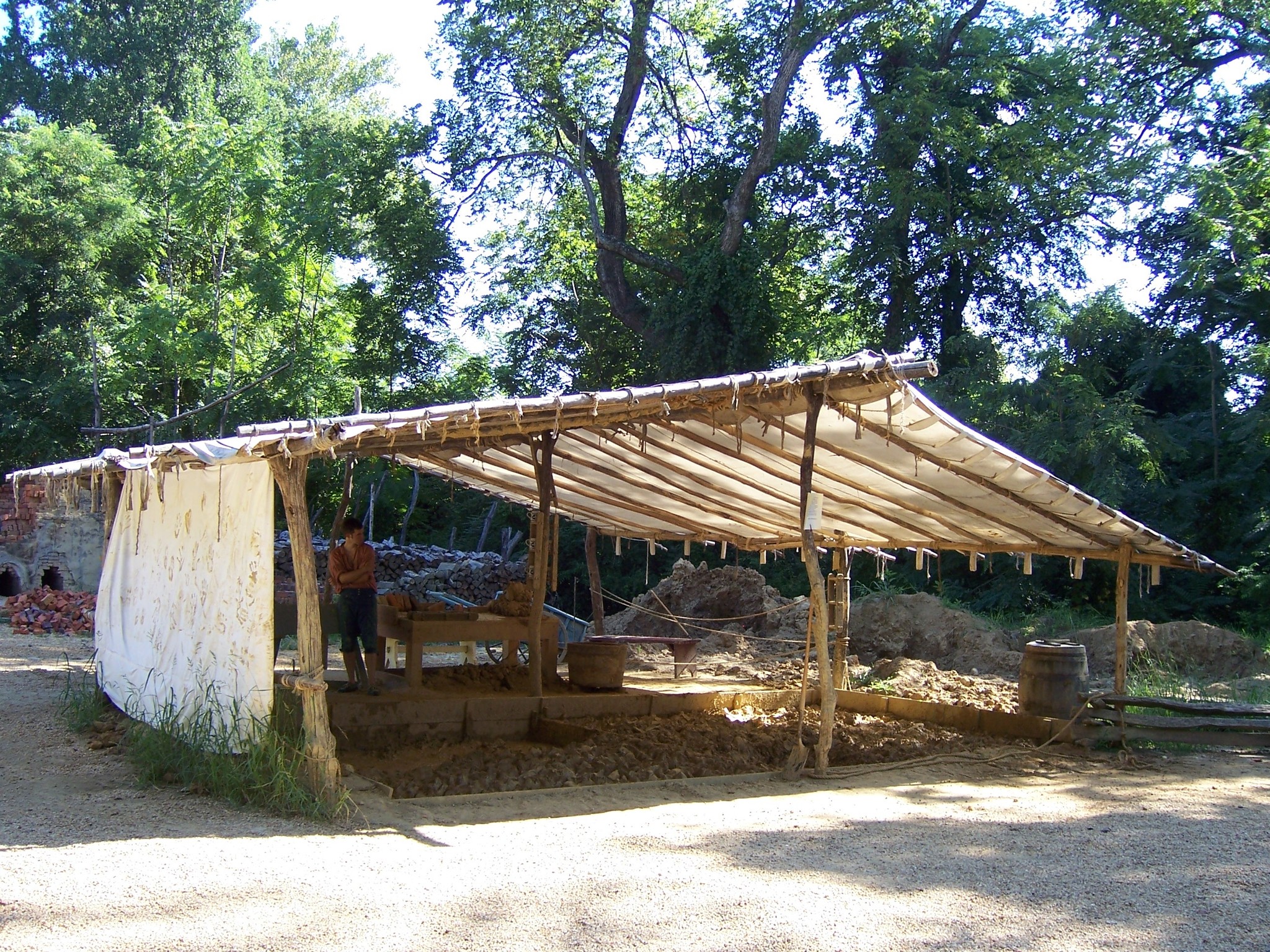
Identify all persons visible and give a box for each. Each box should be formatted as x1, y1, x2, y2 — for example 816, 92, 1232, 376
327, 516, 383, 697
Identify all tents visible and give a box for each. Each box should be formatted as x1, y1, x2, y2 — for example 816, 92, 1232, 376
6, 348, 1231, 803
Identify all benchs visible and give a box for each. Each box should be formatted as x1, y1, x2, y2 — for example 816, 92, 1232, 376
591, 633, 700, 678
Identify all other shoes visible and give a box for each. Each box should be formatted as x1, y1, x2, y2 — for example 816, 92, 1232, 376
367, 684, 380, 695
337, 682, 358, 693
